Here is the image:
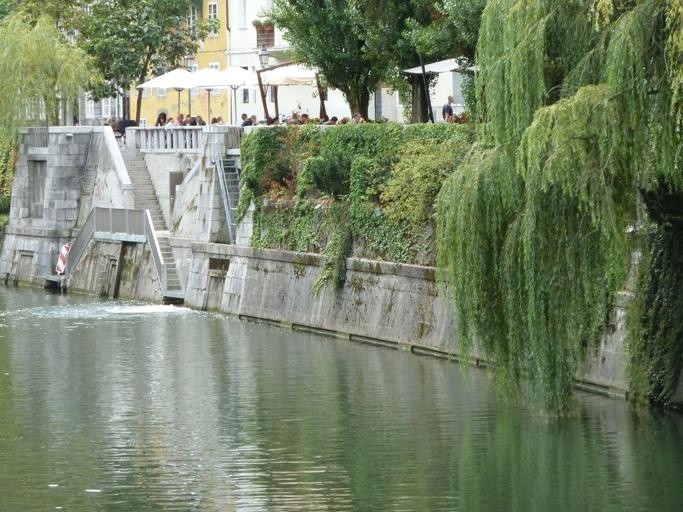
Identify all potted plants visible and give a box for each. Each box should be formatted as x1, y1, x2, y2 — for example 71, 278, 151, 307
253, 20, 263, 31
264, 18, 274, 30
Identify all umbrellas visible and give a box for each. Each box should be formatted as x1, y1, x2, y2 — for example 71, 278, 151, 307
135, 64, 330, 124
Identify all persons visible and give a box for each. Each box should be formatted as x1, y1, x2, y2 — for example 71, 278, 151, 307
103, 117, 122, 138
323, 116, 338, 125
73, 114, 77, 125
353, 112, 366, 123
442, 95, 455, 122
151, 112, 226, 149
241, 113, 309, 127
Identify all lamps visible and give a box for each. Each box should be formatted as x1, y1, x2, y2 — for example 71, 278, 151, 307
258, 44, 273, 68
